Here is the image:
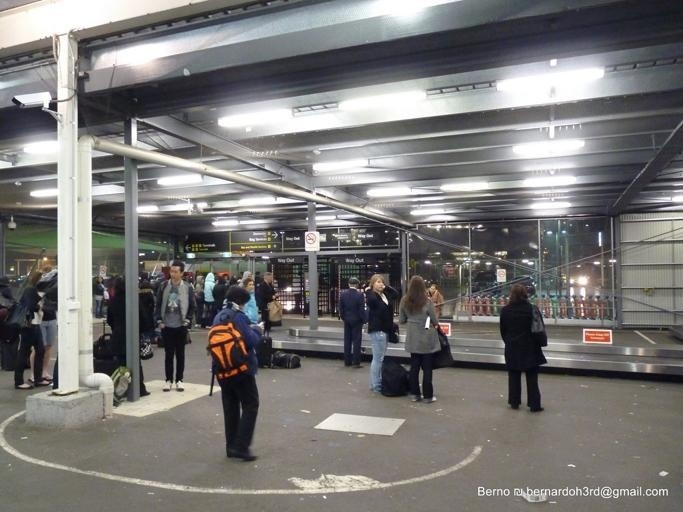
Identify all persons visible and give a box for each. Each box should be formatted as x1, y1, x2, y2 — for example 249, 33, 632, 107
499, 284, 546, 411
337, 271, 443, 403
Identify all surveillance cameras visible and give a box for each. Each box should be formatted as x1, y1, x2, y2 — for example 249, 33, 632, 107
11, 90, 52, 111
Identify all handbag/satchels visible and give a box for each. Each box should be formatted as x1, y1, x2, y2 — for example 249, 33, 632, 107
414, 328, 454, 371
272, 350, 302, 369
381, 356, 409, 397
389, 333, 400, 344
103, 290, 110, 300
139, 333, 154, 360
5, 303, 29, 329
530, 304, 548, 348
266, 299, 283, 322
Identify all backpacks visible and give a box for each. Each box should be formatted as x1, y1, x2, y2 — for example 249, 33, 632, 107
205, 310, 254, 388
109, 366, 132, 408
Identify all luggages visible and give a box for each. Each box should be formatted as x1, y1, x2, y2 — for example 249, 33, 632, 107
263, 335, 273, 368
93, 332, 112, 377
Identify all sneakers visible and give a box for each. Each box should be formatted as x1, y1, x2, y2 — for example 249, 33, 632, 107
162, 380, 172, 391
226, 440, 258, 462
410, 394, 437, 403
96, 315, 105, 318
510, 403, 520, 410
176, 380, 184, 392
530, 407, 544, 412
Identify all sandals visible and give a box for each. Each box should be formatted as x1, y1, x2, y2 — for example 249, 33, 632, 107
15, 375, 53, 389
